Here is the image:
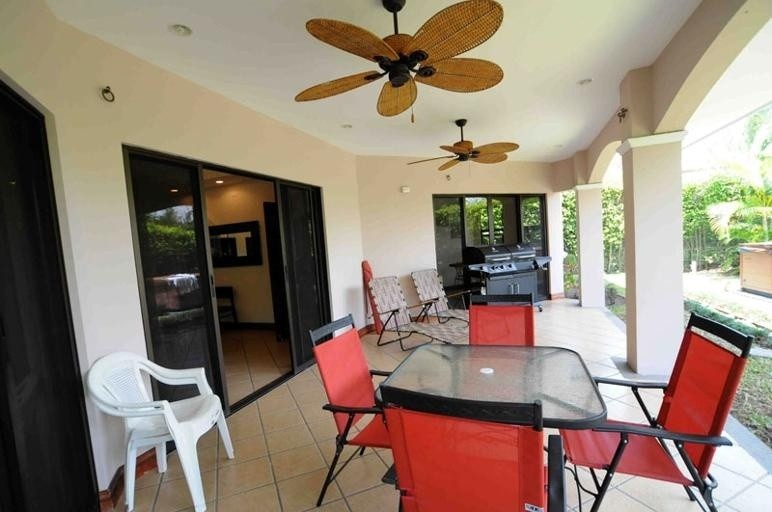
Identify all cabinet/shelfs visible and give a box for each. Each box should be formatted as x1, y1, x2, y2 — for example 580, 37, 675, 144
487, 272, 537, 305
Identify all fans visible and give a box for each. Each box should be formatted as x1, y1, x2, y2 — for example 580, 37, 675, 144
295, 0, 505, 116
407, 118, 520, 171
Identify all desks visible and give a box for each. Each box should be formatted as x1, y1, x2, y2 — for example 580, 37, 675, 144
380, 345, 607, 430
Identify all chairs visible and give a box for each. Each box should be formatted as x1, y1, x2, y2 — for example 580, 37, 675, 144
381, 385, 565, 512
310, 313, 394, 507
369, 268, 473, 351
560, 310, 754, 512
215, 286, 241, 330
468, 292, 535, 347
87, 351, 233, 512
